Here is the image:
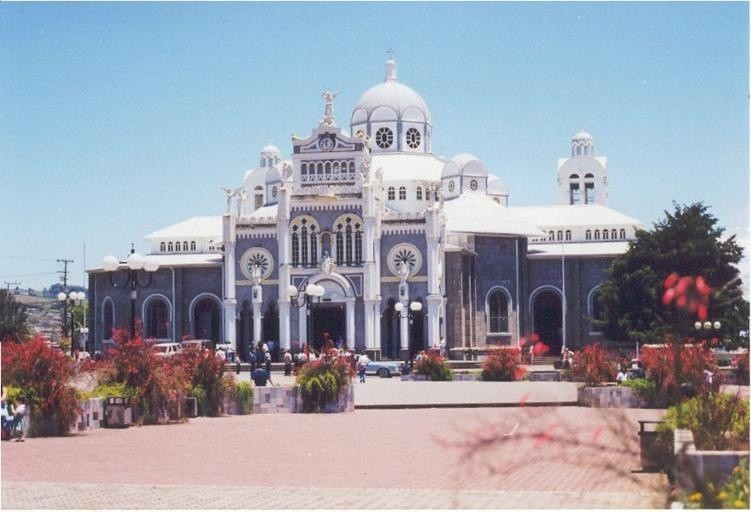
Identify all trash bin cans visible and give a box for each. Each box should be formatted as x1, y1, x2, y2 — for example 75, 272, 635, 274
637, 421, 666, 470
104, 396, 132, 428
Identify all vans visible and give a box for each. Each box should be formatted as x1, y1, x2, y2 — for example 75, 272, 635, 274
149, 339, 215, 361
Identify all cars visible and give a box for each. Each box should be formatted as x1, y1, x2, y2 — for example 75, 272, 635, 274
74, 327, 89, 351
342, 353, 400, 378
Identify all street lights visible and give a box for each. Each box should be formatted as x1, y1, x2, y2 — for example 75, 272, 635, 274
102, 243, 159, 340
694, 320, 722, 348
284, 283, 325, 362
394, 301, 422, 370
58, 291, 85, 357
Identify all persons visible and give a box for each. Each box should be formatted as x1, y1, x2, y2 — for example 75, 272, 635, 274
413, 350, 421, 360
616, 361, 645, 387
226, 189, 232, 213
357, 351, 369, 383
10, 396, 25, 442
1, 385, 9, 440
440, 337, 445, 358
562, 347, 576, 368
215, 336, 343, 386
281, 163, 286, 188
321, 93, 333, 115
